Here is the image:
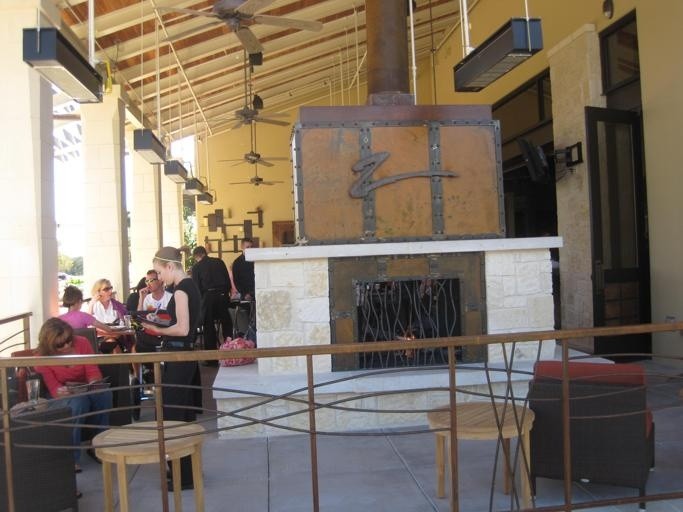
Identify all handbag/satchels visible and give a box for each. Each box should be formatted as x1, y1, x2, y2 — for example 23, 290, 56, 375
219, 337, 255, 367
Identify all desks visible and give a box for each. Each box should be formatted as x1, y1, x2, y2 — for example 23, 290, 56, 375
102, 328, 137, 341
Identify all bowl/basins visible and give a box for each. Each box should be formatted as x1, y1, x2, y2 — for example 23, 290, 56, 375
108, 325, 125, 332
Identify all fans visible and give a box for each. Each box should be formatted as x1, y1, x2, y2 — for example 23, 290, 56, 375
224, 118, 290, 190
163, 0, 323, 60
218, 76, 291, 173
214, 55, 290, 137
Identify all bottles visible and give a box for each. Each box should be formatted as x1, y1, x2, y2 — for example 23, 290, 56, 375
119, 319, 124, 326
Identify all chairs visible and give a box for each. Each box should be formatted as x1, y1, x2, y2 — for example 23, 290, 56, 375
520, 357, 657, 510
71, 325, 96, 352
0, 405, 77, 511
84, 355, 140, 443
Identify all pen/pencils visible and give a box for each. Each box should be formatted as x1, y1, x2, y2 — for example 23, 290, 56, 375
155, 303, 161, 314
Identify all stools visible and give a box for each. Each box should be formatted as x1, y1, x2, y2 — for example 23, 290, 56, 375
427, 401, 536, 512
92, 419, 207, 511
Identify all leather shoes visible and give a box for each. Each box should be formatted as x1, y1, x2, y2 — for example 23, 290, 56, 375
201, 361, 218, 367
166, 480, 194, 492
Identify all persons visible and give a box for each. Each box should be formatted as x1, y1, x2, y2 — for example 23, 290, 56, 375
55, 285, 121, 335
125, 277, 147, 312
35, 316, 112, 472
231, 238, 255, 300
191, 246, 234, 367
151, 245, 204, 489
85, 278, 133, 369
136, 269, 173, 312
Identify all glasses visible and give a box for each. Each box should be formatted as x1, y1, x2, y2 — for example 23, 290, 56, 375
54, 335, 72, 348
144, 278, 159, 283
100, 286, 114, 292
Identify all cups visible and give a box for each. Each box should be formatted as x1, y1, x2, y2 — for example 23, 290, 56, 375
124, 315, 132, 330
25, 373, 42, 409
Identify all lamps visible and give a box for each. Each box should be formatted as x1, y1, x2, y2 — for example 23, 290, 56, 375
444, 0, 544, 94
21, 0, 213, 206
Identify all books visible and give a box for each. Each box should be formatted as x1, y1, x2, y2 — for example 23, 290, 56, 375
62, 374, 111, 393
135, 314, 169, 330
